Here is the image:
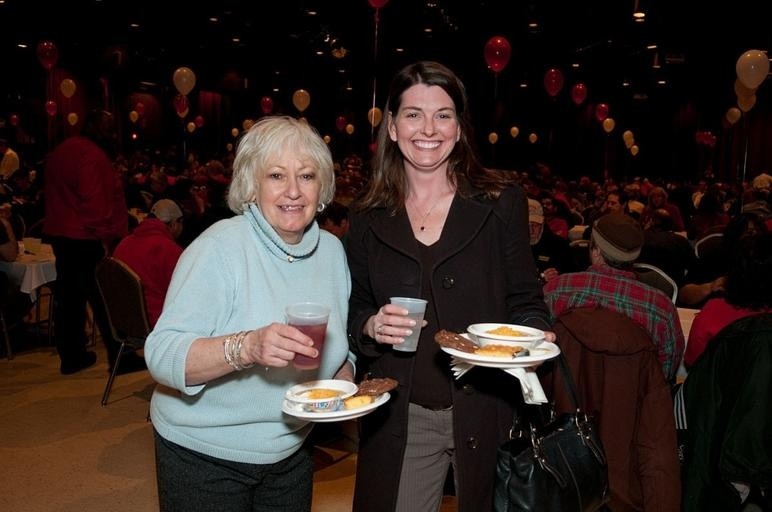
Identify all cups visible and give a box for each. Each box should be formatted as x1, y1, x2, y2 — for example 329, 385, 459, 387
388, 297, 428, 352
283, 301, 329, 370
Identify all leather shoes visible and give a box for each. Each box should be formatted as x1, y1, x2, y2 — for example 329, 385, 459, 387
107, 356, 148, 374
60, 350, 98, 375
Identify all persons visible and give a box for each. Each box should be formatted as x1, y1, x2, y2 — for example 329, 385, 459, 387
2, 112, 233, 375
143, 165, 771, 511
344, 61, 556, 511
144, 114, 354, 512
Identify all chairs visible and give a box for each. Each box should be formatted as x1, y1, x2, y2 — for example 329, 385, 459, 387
694, 233, 725, 258
633, 263, 678, 305
554, 302, 652, 511
95, 256, 151, 406
688, 312, 770, 512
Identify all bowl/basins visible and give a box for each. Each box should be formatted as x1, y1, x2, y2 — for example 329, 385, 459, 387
284, 379, 359, 404
466, 322, 547, 352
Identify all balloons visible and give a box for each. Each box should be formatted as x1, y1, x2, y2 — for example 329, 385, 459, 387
12, 55, 639, 157
484, 36, 512, 72
368, 0, 387, 8
38, 41, 58, 70
725, 50, 769, 124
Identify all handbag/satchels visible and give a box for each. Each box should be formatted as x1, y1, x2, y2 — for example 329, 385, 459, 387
494, 408, 611, 512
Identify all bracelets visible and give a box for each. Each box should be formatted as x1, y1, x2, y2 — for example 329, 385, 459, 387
223, 331, 259, 372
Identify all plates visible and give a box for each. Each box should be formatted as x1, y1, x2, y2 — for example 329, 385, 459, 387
282, 392, 391, 422
440, 332, 561, 368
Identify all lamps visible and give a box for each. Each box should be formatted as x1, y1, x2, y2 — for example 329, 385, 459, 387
633, 0, 646, 18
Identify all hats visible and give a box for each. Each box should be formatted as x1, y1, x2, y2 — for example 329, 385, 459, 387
591, 211, 646, 262
150, 197, 184, 223
525, 198, 546, 224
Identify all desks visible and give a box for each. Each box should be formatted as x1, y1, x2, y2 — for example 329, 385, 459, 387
1, 238, 57, 360
